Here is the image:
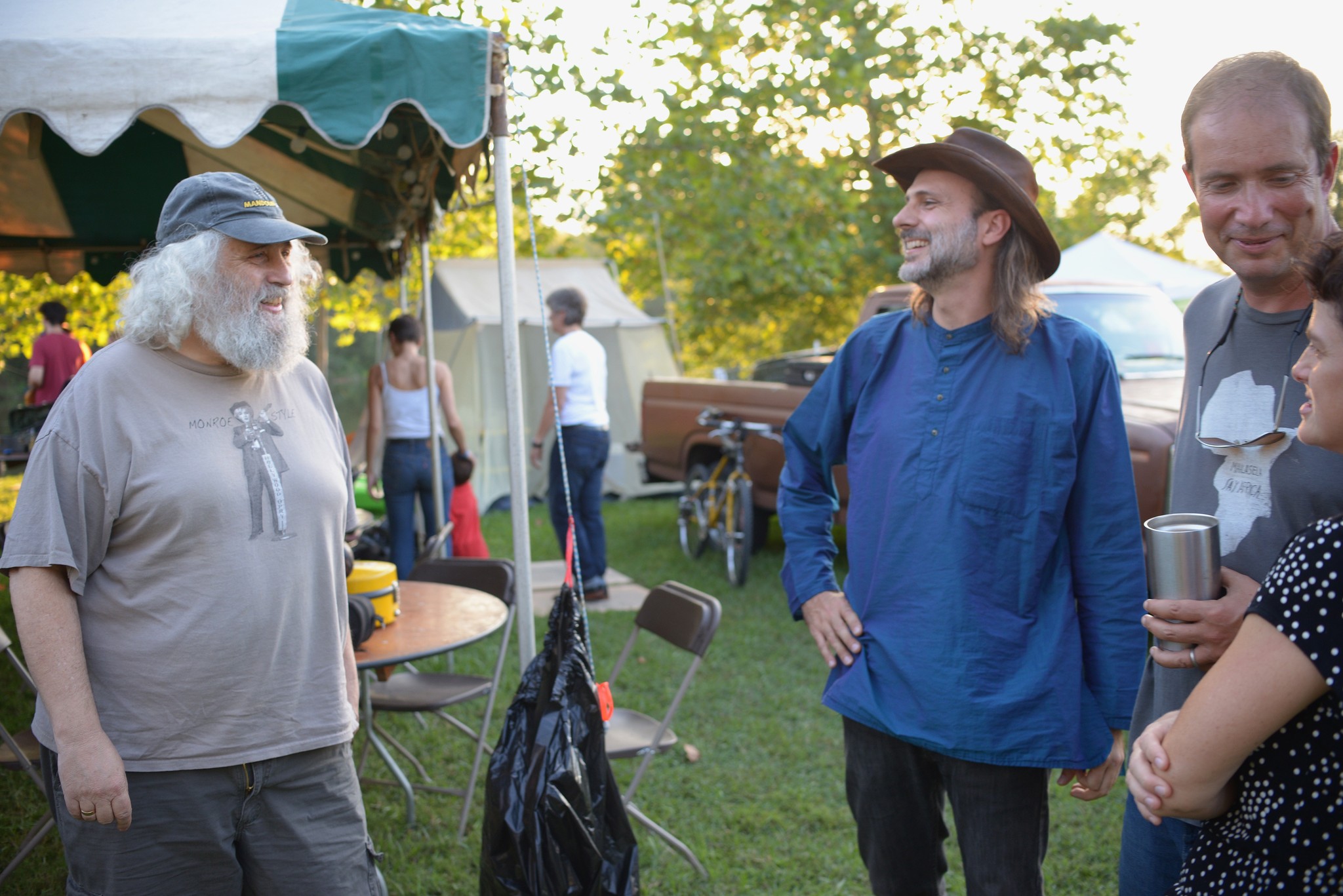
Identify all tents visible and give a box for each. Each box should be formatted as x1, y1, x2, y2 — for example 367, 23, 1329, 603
0, 0, 535, 682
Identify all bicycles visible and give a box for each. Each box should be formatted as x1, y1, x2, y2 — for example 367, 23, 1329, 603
674, 412, 792, 588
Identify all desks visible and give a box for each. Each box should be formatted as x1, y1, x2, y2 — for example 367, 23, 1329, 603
354, 580, 509, 825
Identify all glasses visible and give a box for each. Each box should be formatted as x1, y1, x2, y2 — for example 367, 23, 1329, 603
1194, 331, 1297, 448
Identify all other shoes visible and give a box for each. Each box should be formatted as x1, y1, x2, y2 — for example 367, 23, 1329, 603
553, 582, 610, 602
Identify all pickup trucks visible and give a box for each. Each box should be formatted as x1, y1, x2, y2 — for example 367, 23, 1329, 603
638, 286, 1185, 555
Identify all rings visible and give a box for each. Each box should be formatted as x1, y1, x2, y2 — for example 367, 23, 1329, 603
80, 808, 97, 817
1188, 647, 1201, 670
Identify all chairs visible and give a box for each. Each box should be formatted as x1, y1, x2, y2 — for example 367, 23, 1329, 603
357, 558, 517, 844
604, 580, 723, 879
0, 627, 56, 883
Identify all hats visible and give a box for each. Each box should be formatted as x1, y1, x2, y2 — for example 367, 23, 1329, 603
155, 171, 327, 245
871, 125, 1060, 283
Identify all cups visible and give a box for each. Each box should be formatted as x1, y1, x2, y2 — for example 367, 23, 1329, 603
1143, 512, 1221, 653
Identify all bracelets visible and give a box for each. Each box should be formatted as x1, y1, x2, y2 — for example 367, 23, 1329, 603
532, 439, 544, 450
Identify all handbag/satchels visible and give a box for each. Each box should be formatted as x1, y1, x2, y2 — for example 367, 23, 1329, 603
451, 480, 492, 559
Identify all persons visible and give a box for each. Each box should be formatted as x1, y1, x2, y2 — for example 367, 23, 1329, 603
1118, 50, 1343, 896
228, 401, 291, 543
0, 171, 389, 896
775, 126, 1158, 896
529, 288, 612, 602
22, 302, 87, 452
1125, 228, 1343, 896
367, 313, 488, 580
